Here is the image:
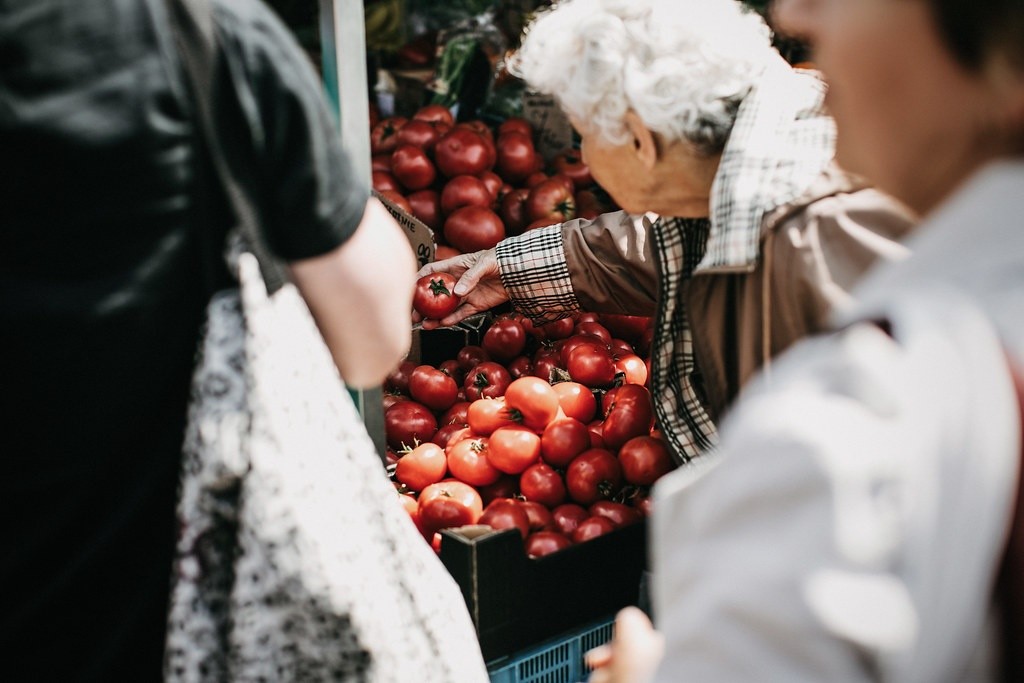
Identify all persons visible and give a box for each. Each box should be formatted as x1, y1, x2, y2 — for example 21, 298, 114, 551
410, 0, 928, 466
0, 0, 418, 683
585, 0, 1024, 683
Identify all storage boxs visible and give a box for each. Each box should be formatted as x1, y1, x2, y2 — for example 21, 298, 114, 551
438, 516, 650, 683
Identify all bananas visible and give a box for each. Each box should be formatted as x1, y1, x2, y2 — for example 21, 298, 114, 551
364, 0, 408, 52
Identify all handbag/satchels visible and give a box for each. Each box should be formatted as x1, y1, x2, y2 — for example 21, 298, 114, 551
153, 1, 492, 683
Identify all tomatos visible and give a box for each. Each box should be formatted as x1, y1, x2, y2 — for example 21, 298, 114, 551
382, 307, 674, 558
368, 105, 616, 316
